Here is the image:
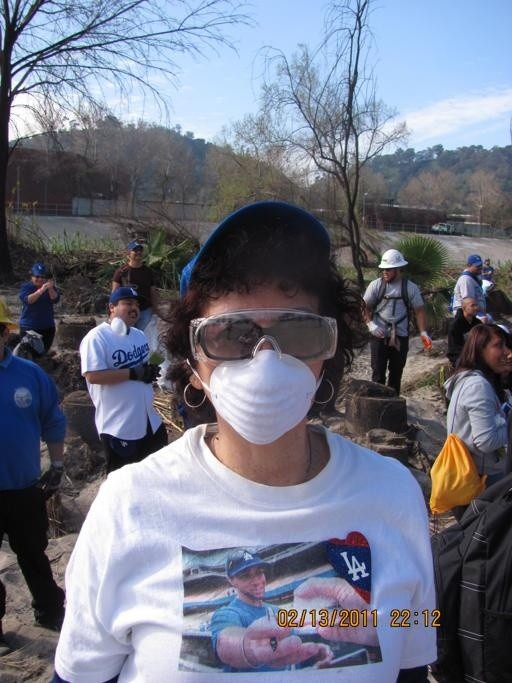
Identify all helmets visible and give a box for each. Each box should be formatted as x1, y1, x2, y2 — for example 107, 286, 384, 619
1, 296, 19, 332
378, 249, 408, 270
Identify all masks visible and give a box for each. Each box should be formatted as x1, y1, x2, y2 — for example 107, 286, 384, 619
185, 350, 328, 447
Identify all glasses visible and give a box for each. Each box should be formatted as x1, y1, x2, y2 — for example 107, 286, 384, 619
196, 317, 334, 361
189, 308, 338, 367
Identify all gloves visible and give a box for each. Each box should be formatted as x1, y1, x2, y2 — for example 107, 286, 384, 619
421, 331, 433, 352
35, 467, 63, 504
366, 320, 385, 340
129, 363, 161, 385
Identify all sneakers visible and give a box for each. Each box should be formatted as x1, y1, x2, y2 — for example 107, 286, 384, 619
0, 619, 10, 657
31, 607, 65, 634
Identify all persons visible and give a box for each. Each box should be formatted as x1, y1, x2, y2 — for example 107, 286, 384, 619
447, 298, 483, 362
207, 546, 317, 671
292, 576, 376, 644
78, 284, 168, 471
20, 261, 62, 353
445, 322, 511, 522
49, 200, 438, 683
110, 235, 159, 333
0, 297, 67, 636
451, 253, 488, 322
482, 255, 494, 282
360, 246, 432, 394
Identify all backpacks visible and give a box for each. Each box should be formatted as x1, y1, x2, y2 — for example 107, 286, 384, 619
429, 375, 489, 515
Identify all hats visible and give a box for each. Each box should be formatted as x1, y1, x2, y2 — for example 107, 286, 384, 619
176, 198, 332, 300
468, 253, 483, 267
126, 240, 144, 251
108, 286, 142, 306
223, 548, 272, 579
29, 263, 49, 279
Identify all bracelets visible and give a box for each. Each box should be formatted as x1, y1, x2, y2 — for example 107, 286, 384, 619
240, 637, 266, 670
